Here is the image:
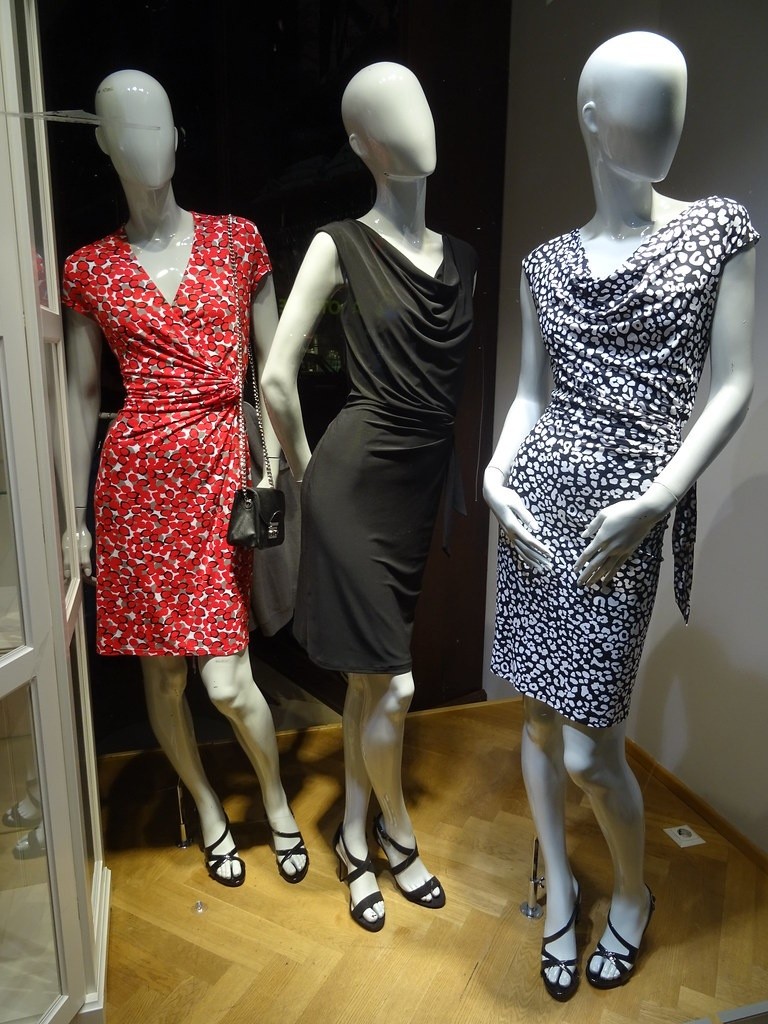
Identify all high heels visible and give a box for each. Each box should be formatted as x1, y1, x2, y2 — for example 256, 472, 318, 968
332, 822, 386, 933
540, 875, 582, 1002
585, 882, 656, 990
265, 798, 309, 883
372, 811, 446, 909
198, 805, 246, 887
3, 779, 43, 827
13, 828, 47, 859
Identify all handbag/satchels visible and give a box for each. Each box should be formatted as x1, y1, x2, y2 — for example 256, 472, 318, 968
226, 486, 286, 550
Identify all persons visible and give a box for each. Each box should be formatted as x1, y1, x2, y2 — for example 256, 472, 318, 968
260, 58, 480, 933
482, 29, 761, 1004
62, 69, 309, 889
